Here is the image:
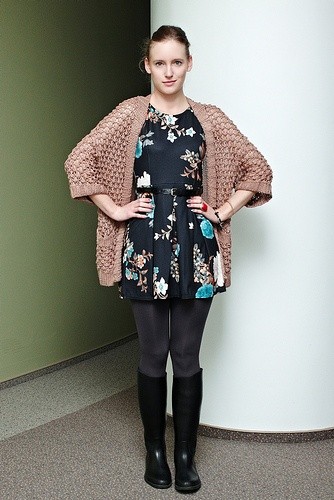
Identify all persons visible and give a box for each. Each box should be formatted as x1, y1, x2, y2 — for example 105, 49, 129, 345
63, 25, 272, 493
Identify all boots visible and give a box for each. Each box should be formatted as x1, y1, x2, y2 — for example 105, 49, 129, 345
173, 369, 202, 494
137, 366, 172, 489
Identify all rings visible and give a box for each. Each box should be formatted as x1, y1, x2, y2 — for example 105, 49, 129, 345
201, 203, 208, 212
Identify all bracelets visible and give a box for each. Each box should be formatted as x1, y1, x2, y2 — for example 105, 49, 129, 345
214, 211, 222, 223
226, 201, 234, 215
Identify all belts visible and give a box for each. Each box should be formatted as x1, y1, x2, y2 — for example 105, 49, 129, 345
134, 187, 200, 197
